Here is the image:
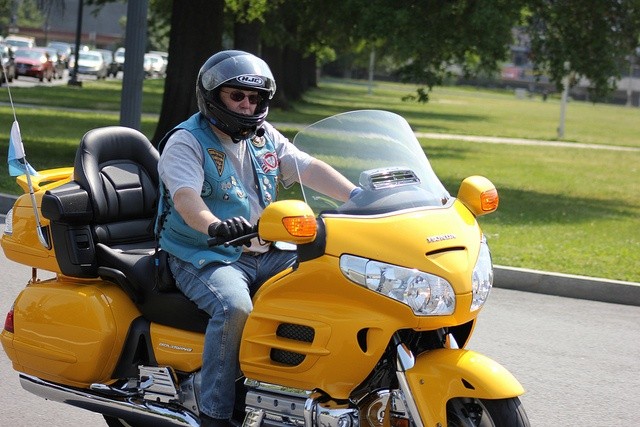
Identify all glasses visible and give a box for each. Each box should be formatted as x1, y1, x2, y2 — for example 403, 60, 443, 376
221, 91, 264, 105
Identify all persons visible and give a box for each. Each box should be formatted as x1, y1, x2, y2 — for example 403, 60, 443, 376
153, 49, 372, 425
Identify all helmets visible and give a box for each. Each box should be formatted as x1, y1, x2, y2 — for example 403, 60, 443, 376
195, 49, 277, 140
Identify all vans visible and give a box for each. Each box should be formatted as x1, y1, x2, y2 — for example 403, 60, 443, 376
4, 33, 36, 55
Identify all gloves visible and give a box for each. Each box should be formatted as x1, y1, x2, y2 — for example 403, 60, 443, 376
350, 187, 375, 207
208, 216, 253, 247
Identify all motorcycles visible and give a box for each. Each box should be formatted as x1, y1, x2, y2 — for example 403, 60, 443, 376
0, 57, 531, 426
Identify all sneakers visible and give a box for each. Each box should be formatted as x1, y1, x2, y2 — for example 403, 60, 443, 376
200, 413, 236, 427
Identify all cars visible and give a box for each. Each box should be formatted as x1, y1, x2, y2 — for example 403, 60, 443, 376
144, 51, 168, 77
100, 48, 115, 77
114, 47, 125, 71
11, 46, 53, 80
49, 49, 65, 79
0, 37, 15, 84
48, 42, 71, 69
68, 49, 107, 79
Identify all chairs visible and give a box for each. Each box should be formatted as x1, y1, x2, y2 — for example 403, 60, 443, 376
41, 124, 210, 335
